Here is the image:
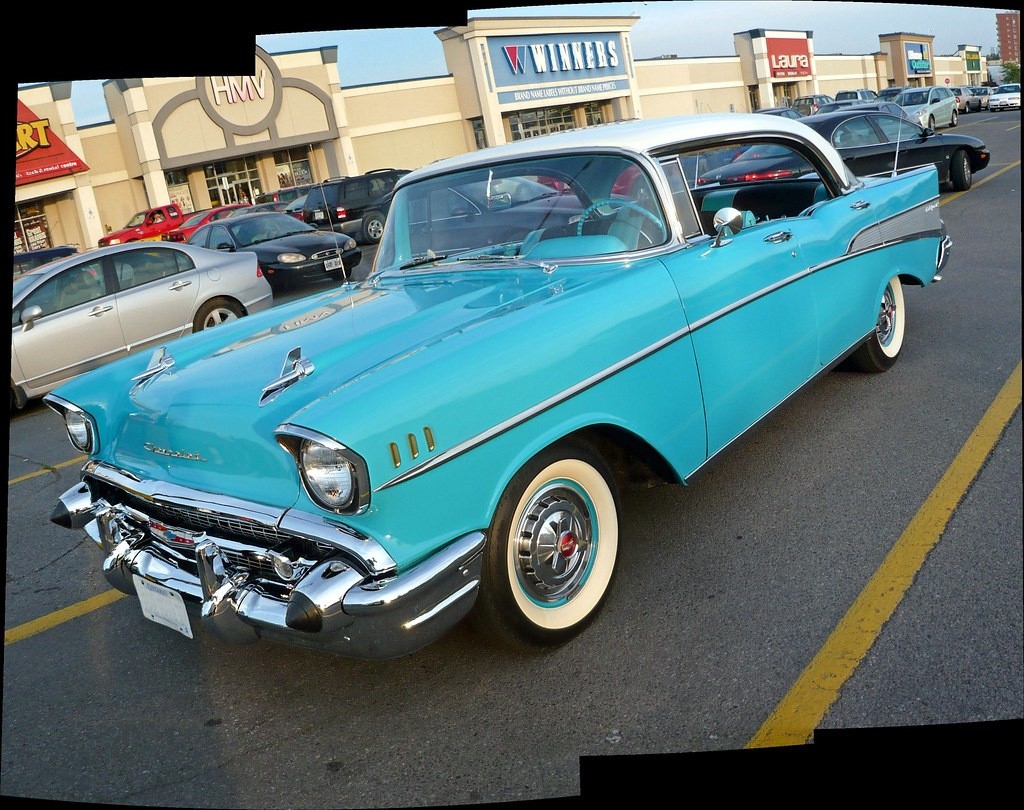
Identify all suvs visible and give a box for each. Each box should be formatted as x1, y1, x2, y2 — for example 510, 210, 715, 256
792, 94, 835, 116
836, 89, 877, 103
256, 184, 316, 204
303, 168, 413, 243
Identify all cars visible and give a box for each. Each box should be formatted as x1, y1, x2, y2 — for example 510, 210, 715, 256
696, 108, 991, 190
13, 246, 77, 281
835, 103, 922, 144
43, 113, 951, 646
185, 212, 363, 289
948, 87, 982, 113
892, 86, 959, 133
755, 107, 807, 120
877, 87, 913, 103
226, 202, 289, 217
284, 194, 309, 223
162, 204, 252, 244
814, 100, 867, 115
408, 178, 628, 256
12, 240, 274, 415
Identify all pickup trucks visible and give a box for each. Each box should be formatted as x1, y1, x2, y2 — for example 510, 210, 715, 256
96, 204, 217, 261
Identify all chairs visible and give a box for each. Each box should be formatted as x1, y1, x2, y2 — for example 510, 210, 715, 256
517, 224, 577, 256
607, 176, 669, 250
71, 272, 102, 305
840, 130, 860, 146
59, 278, 78, 309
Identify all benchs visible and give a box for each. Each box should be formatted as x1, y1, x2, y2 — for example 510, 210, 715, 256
619, 181, 828, 237
132, 260, 184, 288
